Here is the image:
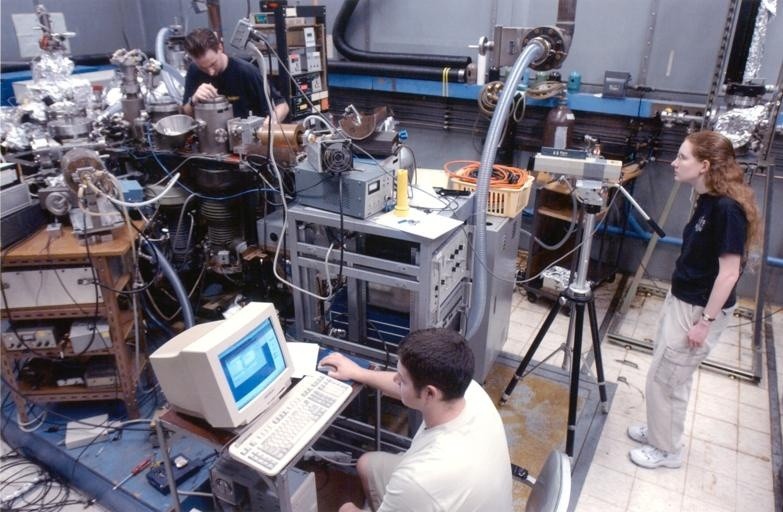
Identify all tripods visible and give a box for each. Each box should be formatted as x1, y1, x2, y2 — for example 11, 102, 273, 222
498, 178, 665, 463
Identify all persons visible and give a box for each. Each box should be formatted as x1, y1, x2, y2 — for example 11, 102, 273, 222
625, 129, 764, 470
320, 326, 515, 512
179, 26, 290, 129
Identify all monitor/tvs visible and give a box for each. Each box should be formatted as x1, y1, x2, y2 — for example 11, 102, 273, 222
148, 301, 293, 435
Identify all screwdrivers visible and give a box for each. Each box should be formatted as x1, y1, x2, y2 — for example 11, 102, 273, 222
112, 457, 152, 491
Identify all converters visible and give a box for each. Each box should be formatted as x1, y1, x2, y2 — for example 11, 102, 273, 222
145, 452, 199, 495
64, 413, 110, 448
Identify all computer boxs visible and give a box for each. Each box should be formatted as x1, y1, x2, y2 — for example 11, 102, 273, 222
208, 454, 318, 512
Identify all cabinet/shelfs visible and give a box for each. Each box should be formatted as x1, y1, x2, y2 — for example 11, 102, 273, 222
1, 217, 167, 433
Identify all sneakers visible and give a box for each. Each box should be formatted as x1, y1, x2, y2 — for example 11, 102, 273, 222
625, 424, 649, 444
629, 445, 683, 469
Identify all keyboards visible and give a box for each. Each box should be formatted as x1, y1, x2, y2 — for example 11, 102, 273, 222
227, 370, 353, 476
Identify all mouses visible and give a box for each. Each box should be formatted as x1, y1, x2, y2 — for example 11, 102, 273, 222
317, 361, 336, 372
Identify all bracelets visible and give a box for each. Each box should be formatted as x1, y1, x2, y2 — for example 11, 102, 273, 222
702, 311, 717, 323
187, 94, 194, 106
